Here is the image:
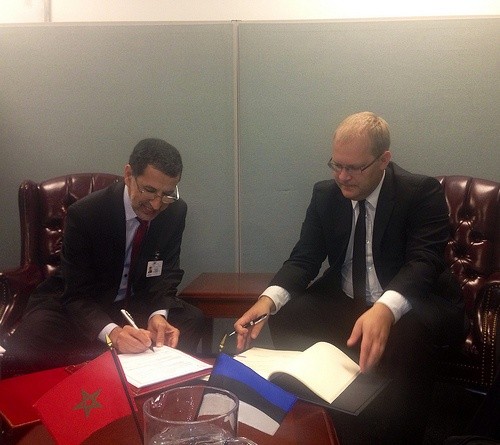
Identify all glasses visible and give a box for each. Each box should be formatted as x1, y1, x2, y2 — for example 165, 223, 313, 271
130, 166, 180, 204
327, 151, 383, 177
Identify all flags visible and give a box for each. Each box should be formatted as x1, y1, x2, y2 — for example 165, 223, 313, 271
198, 351, 297, 437
32, 350, 139, 445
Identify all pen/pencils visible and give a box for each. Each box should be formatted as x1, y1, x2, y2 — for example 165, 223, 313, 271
120, 309, 155, 352
228, 313, 268, 336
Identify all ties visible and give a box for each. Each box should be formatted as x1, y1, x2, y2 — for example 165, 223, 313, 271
351, 199, 367, 320
119, 217, 149, 327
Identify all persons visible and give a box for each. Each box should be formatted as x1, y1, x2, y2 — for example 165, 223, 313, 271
4, 139, 207, 378
230, 111, 466, 445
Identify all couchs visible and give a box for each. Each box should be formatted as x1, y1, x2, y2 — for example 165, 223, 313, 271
0, 171, 122, 341
428, 174, 500, 401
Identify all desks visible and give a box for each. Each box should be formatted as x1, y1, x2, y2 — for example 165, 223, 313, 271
2, 362, 345, 444
182, 270, 278, 357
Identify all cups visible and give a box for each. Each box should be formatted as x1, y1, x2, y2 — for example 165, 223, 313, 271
143, 386, 258, 445
